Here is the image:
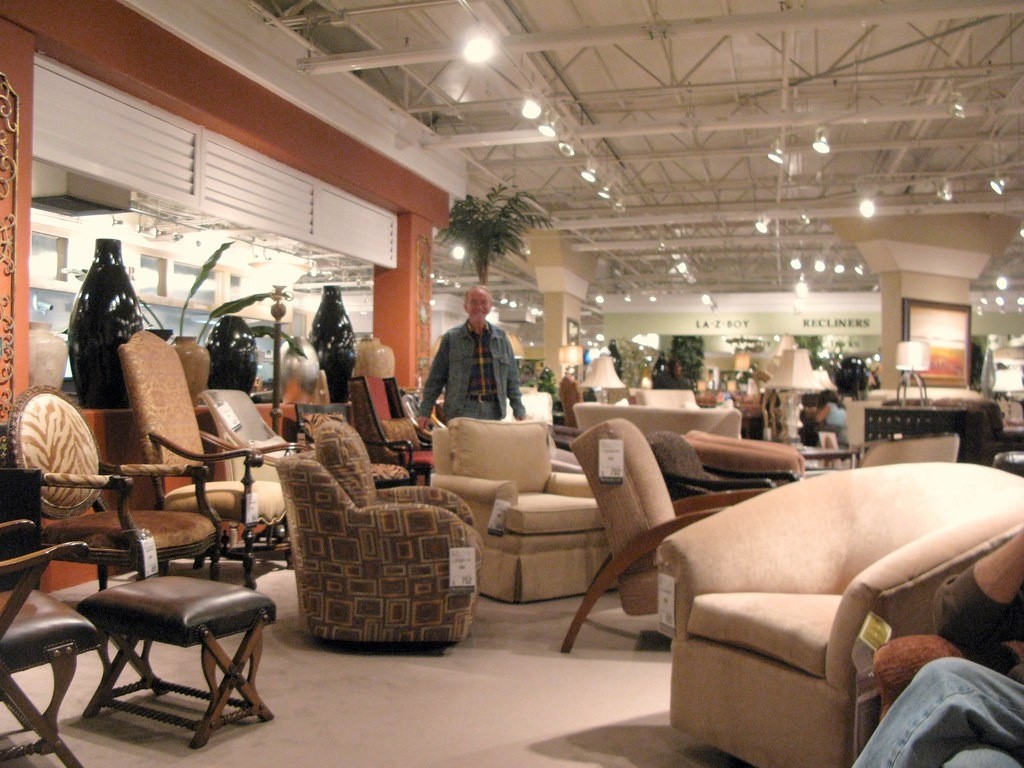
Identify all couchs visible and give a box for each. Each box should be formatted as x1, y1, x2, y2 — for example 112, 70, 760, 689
277, 384, 1024, 768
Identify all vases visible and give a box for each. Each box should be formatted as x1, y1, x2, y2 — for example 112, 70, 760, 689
27, 238, 395, 409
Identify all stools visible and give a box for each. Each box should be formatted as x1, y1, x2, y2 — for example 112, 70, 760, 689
78, 576, 276, 751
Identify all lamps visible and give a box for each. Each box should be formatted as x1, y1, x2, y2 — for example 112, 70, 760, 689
895, 340, 933, 407
418, 36, 1024, 315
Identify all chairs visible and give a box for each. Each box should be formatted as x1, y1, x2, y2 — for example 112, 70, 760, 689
560, 420, 774, 653
0, 329, 434, 768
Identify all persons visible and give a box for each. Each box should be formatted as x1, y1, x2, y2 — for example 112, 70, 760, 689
853, 528, 1024, 768
418, 286, 526, 435
797, 389, 847, 447
661, 355, 690, 390
